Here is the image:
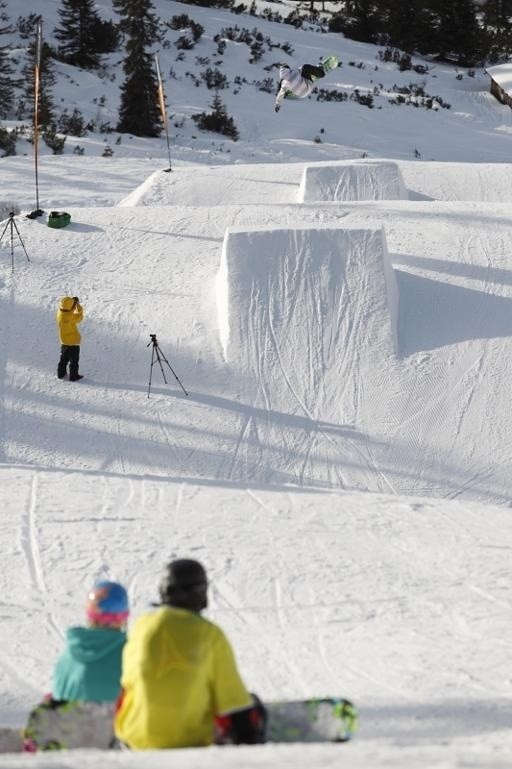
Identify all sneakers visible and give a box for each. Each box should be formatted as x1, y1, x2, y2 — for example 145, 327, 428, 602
58, 371, 83, 381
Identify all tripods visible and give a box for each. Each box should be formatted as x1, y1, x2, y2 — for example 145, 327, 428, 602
145, 334, 189, 398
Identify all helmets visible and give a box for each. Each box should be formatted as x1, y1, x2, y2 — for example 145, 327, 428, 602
86, 580, 129, 625
158, 559, 206, 610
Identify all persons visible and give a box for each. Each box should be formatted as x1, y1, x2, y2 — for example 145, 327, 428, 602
54, 580, 130, 702
56, 295, 87, 380
110, 558, 268, 748
273, 62, 326, 113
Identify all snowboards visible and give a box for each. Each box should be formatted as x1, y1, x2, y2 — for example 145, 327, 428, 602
25, 694, 356, 749
